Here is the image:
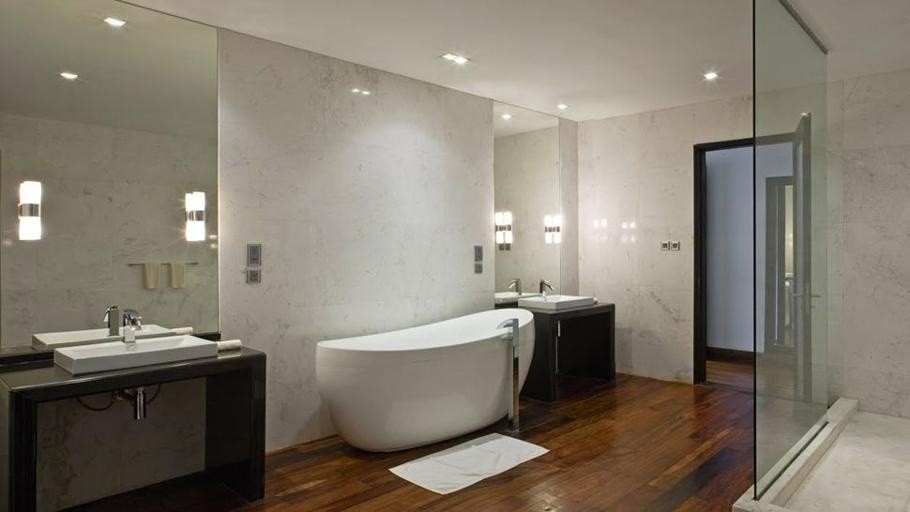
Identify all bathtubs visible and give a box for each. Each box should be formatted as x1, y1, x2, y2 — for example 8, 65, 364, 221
313, 307, 536, 452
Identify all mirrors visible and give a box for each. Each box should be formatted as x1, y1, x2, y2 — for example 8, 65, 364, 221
493, 101, 561, 307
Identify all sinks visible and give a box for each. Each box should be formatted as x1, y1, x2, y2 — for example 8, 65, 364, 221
495, 291, 542, 305
53, 334, 219, 374
517, 294, 593, 310
31, 324, 175, 352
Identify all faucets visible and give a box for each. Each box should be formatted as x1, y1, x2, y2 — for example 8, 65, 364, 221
507, 278, 522, 296
539, 278, 555, 294
122, 309, 142, 341
102, 305, 121, 337
496, 318, 520, 435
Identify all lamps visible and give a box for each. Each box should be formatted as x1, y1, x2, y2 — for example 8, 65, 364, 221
18, 181, 42, 241
502, 212, 513, 243
545, 215, 552, 244
495, 213, 504, 244
553, 215, 560, 244
0, 0, 220, 365
184, 190, 206, 242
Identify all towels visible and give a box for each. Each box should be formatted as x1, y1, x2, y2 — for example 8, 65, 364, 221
169, 261, 187, 289
143, 261, 161, 290
388, 432, 552, 496
